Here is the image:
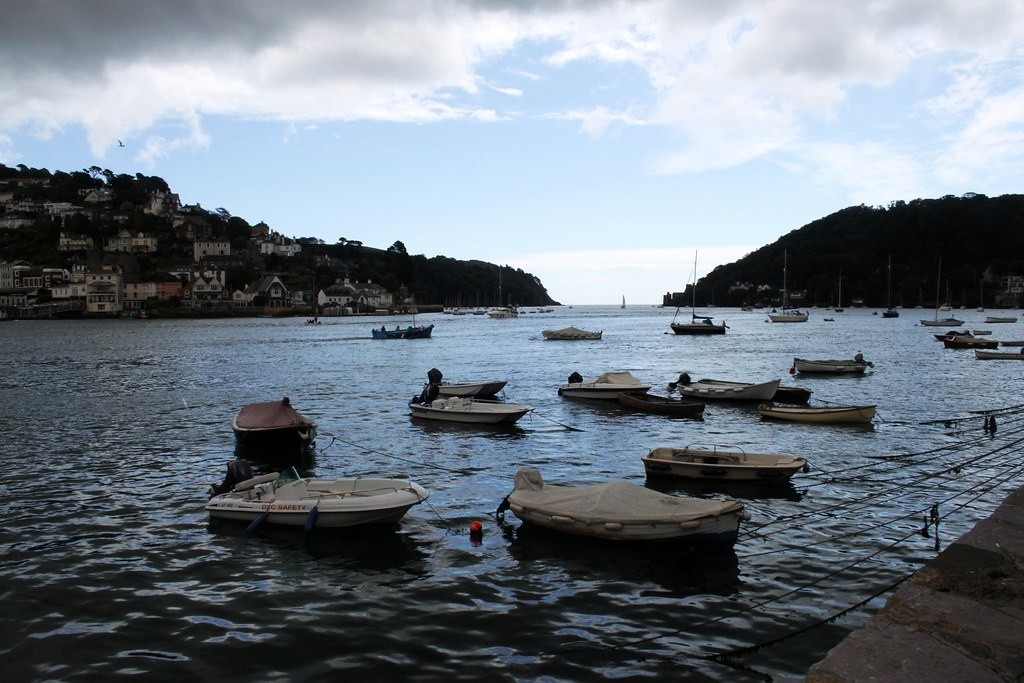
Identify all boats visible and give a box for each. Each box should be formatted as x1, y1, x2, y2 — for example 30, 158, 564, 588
666, 372, 781, 406
407, 384, 535, 428
771, 386, 814, 405
541, 326, 603, 340
756, 400, 878, 425
230, 396, 319, 468
423, 367, 508, 399
617, 390, 706, 419
789, 351, 875, 374
919, 255, 1024, 361
371, 322, 435, 340
557, 371, 653, 402
204, 457, 431, 535
494, 466, 752, 563
640, 439, 811, 487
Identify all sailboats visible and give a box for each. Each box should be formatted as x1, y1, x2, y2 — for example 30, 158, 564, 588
669, 250, 729, 335
834, 269, 844, 313
881, 252, 900, 318
767, 248, 810, 323
485, 264, 520, 320
